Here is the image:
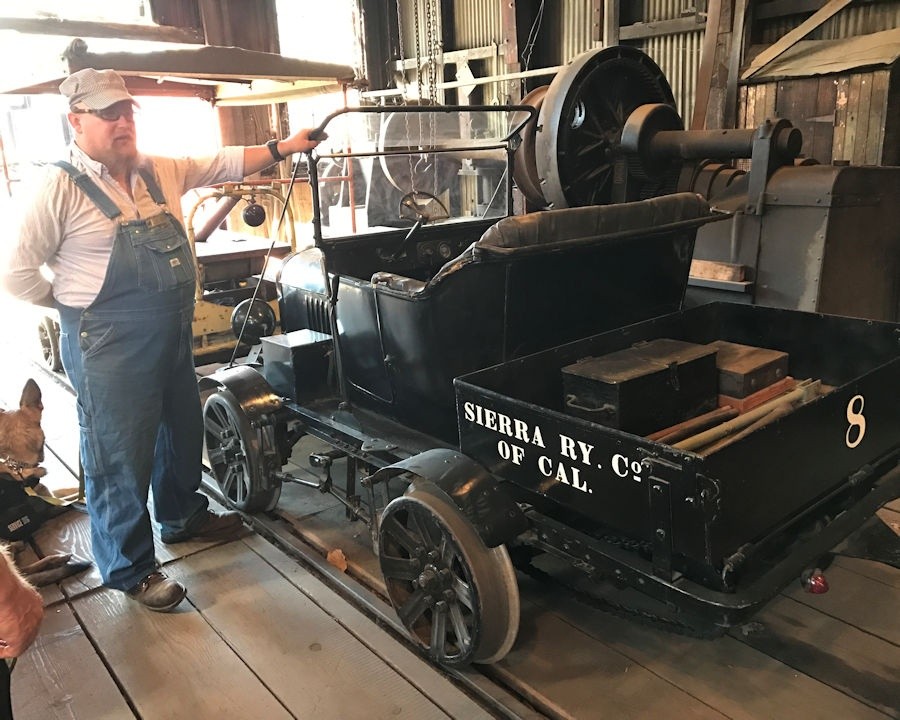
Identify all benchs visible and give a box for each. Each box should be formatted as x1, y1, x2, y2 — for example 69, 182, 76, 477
366, 192, 706, 439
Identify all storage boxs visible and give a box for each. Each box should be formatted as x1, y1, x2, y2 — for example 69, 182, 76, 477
562, 337, 721, 438
702, 338, 791, 415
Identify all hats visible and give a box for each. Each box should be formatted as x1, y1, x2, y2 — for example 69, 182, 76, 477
58, 66, 139, 110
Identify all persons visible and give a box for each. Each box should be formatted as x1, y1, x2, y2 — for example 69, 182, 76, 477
7, 66, 330, 612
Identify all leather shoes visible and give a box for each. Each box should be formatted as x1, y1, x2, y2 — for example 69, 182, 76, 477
184, 511, 241, 536
125, 571, 186, 613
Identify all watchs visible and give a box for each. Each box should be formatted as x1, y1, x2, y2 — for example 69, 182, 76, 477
267, 138, 286, 163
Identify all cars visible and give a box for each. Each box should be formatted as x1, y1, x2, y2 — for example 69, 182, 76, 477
198, 45, 898, 668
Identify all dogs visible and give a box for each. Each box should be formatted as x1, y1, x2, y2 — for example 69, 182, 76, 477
0, 380, 93, 585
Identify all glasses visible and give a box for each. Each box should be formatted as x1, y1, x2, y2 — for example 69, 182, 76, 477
72, 109, 135, 121
